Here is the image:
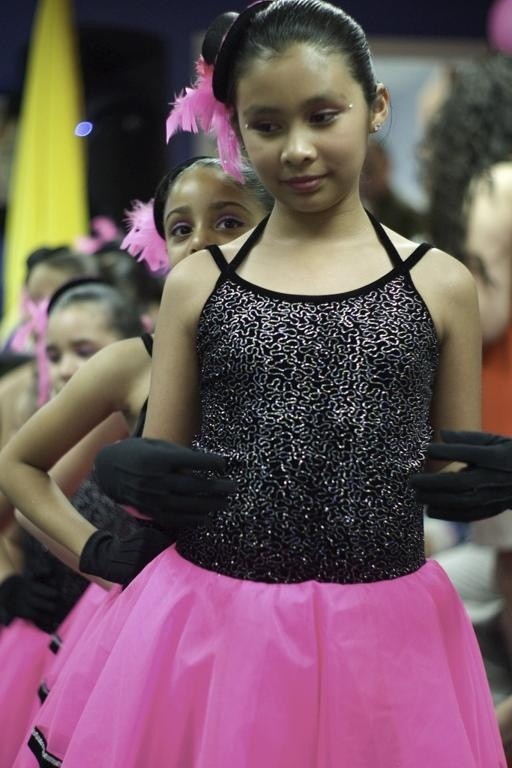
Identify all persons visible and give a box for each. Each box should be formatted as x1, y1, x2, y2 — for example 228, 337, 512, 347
27, 1, 512, 767
410, 51, 511, 662
0, 153, 276, 768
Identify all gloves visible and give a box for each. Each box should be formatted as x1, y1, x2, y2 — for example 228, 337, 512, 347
0, 575, 64, 633
413, 430, 511, 523
79, 527, 163, 580
94, 437, 228, 525
